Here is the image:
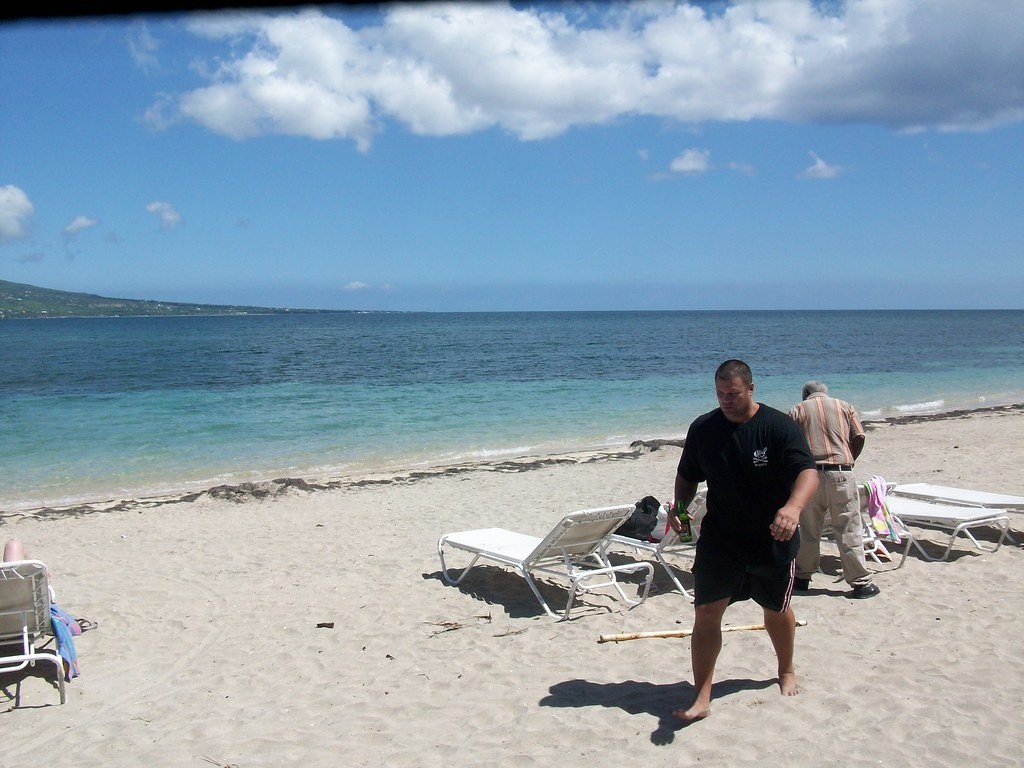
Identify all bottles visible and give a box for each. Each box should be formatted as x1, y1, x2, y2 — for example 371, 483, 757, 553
677, 499, 692, 543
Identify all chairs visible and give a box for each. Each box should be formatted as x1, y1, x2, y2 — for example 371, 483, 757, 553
437, 503, 655, 621
0, 559, 66, 705
590, 487, 708, 600
867, 495, 1010, 562
892, 482, 1024, 546
816, 482, 912, 574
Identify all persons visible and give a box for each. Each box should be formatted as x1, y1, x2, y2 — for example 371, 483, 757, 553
3, 536, 51, 586
788, 379, 881, 597
666, 359, 820, 721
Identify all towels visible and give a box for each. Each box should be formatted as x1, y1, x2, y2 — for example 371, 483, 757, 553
47, 603, 82, 683
863, 473, 902, 545
